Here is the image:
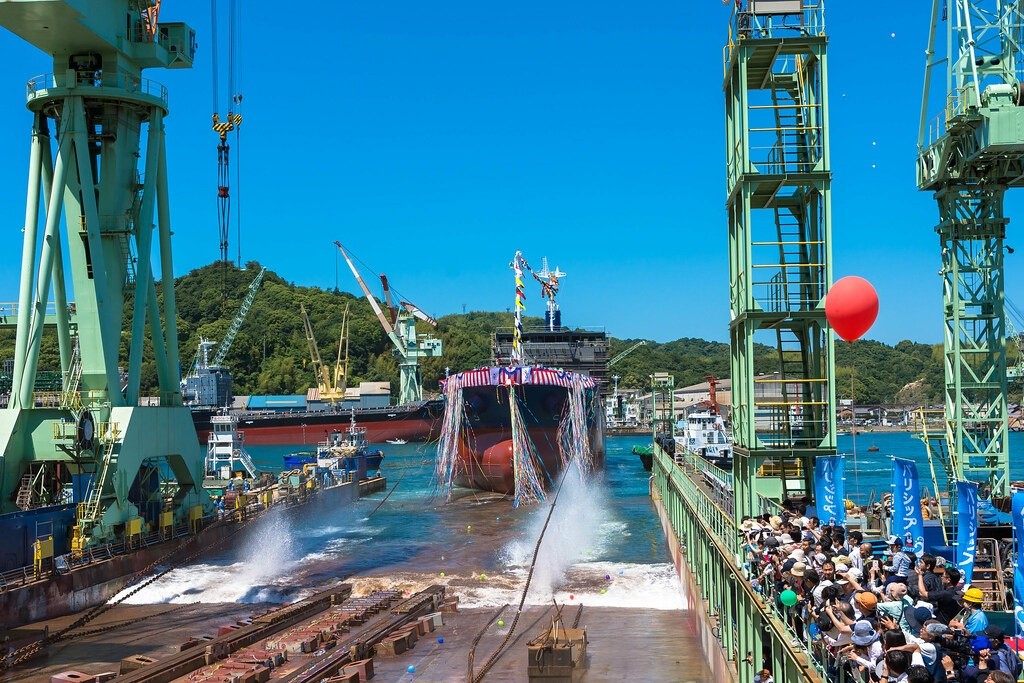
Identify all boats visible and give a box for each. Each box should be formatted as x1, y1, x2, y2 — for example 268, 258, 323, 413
283, 406, 385, 477
632, 404, 765, 470
439, 251, 607, 493
188, 400, 446, 446
526, 601, 588, 683
386, 438, 408, 445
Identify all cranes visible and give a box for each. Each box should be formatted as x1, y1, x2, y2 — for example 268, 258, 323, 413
605, 341, 647, 370
300, 302, 350, 405
210, 267, 267, 367
0, 0, 246, 542
333, 242, 442, 406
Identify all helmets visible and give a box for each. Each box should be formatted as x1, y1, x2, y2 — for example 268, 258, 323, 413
962, 588, 984, 603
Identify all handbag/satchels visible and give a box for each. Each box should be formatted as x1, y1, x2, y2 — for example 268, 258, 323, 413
817, 611, 840, 632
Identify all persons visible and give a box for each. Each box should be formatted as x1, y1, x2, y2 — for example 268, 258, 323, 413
228, 478, 233, 491
219, 497, 225, 517
212, 495, 219, 507
738, 499, 1023, 683
244, 479, 249, 493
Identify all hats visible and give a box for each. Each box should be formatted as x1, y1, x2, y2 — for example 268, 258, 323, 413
976, 625, 1004, 641
855, 592, 877, 610
847, 531, 863, 542
905, 607, 932, 633
780, 549, 807, 577
781, 534, 794, 544
740, 521, 753, 531
889, 583, 907, 600
814, 553, 864, 585
769, 516, 782, 530
913, 601, 936, 618
885, 536, 903, 547
851, 620, 879, 646
775, 536, 783, 546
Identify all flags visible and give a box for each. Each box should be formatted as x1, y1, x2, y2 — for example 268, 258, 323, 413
513, 249, 558, 360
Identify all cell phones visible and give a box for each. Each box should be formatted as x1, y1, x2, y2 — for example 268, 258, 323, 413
873, 560, 878, 570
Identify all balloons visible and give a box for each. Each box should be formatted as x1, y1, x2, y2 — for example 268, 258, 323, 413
825, 276, 879, 343
